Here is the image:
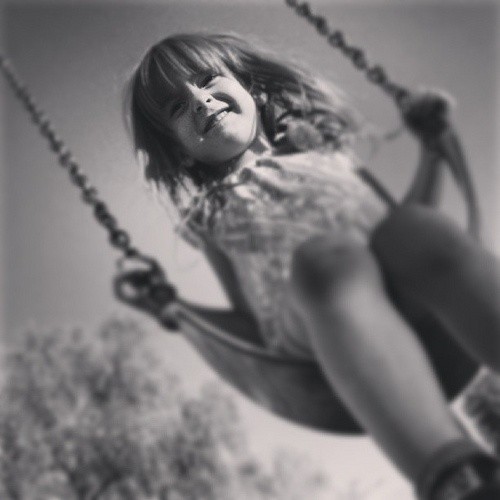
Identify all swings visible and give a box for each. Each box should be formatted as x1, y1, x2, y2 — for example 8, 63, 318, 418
0, 0, 490, 439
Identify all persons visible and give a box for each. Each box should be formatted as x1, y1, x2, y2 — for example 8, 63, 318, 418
113, 22, 500, 500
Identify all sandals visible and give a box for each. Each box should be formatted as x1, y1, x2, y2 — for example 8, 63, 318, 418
415, 439, 499, 499
